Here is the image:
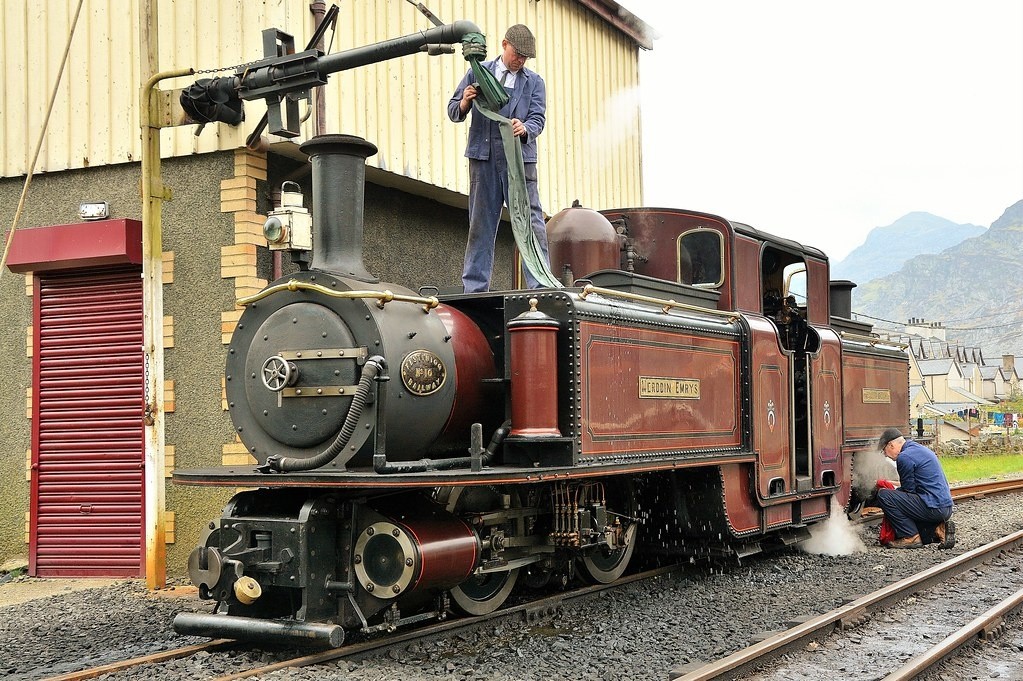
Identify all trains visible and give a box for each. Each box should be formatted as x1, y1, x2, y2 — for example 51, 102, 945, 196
172, 130, 916, 653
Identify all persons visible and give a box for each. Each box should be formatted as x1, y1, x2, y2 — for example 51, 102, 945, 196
877, 428, 955, 549
447, 25, 556, 294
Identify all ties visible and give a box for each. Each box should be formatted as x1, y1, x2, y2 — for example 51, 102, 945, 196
500, 70, 511, 87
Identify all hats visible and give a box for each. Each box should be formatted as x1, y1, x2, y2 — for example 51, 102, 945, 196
877, 428, 903, 451
505, 24, 536, 58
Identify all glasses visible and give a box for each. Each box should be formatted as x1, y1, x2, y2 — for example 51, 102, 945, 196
880, 445, 887, 453
506, 40, 529, 60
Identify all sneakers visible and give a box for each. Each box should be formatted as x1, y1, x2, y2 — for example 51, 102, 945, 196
886, 534, 923, 549
935, 521, 955, 550
